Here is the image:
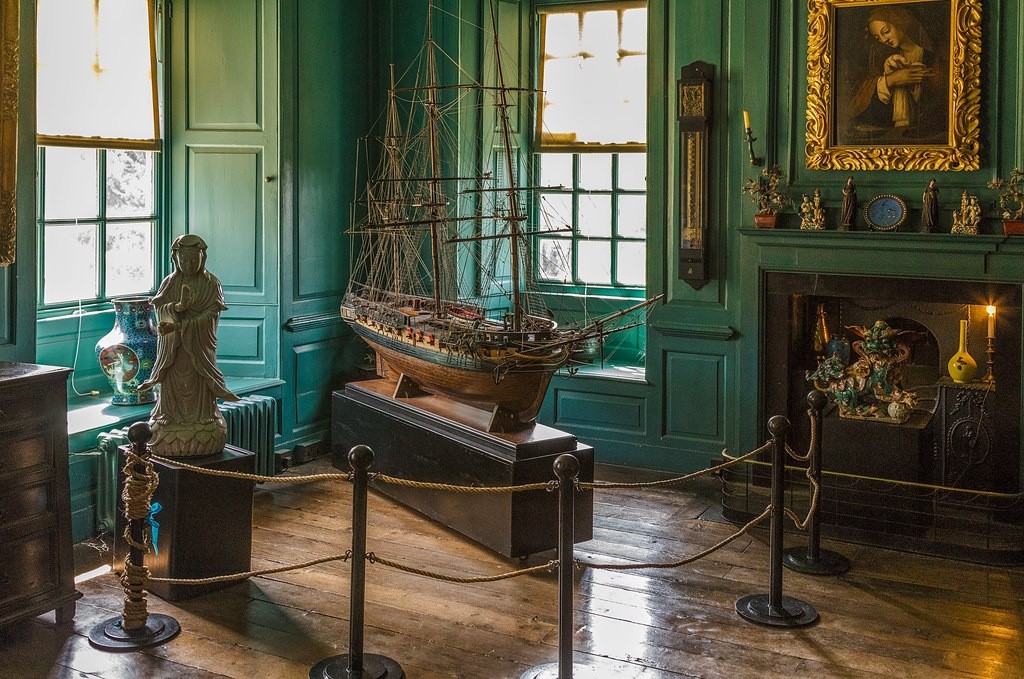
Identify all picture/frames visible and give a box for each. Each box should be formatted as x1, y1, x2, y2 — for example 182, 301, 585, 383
805, 0, 983, 173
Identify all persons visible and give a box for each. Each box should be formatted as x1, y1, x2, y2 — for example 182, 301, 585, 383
800, 187, 825, 224
922, 178, 941, 226
839, 176, 858, 229
953, 191, 982, 227
148, 234, 241, 458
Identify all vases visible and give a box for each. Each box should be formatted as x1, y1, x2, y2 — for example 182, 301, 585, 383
947, 319, 978, 383
95, 295, 157, 406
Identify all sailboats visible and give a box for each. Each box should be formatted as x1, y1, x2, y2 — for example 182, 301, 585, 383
339, 1, 667, 434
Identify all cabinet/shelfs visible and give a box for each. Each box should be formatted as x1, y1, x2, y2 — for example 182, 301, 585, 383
0, 360, 84, 631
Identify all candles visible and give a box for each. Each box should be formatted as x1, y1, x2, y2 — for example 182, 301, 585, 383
743, 110, 750, 129
987, 313, 994, 337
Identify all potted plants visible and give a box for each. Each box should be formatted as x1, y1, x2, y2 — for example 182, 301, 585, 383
986, 166, 1024, 236
741, 162, 789, 229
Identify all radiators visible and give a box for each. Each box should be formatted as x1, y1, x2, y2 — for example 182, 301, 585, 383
95, 394, 277, 535
